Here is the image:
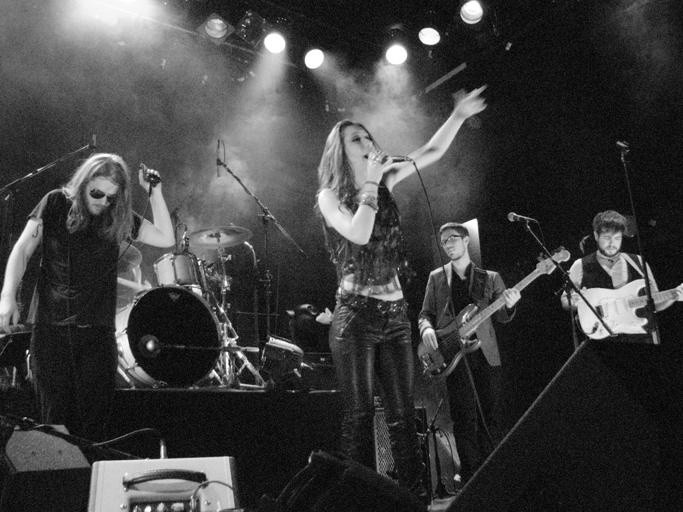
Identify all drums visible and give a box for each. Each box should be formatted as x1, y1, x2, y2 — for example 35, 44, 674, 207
119, 281, 223, 391
151, 248, 203, 289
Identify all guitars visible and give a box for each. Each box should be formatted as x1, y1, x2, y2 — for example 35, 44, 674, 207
576, 276, 682, 342
417, 247, 572, 384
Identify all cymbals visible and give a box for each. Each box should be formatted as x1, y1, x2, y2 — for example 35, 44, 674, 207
186, 225, 254, 251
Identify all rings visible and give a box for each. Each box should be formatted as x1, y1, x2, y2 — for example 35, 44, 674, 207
376, 158, 382, 162
372, 156, 375, 160
368, 156, 371, 159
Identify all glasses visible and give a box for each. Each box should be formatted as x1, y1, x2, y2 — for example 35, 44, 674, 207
89, 182, 118, 205
440, 234, 465, 246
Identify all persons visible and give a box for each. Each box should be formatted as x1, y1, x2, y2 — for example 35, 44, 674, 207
418, 221, 520, 488
313, 85, 487, 505
560, 209, 662, 345
0, 152, 176, 443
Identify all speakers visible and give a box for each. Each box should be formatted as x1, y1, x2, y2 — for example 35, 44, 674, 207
373, 406, 431, 504
260, 452, 427, 511
88, 457, 247, 512
3, 424, 90, 511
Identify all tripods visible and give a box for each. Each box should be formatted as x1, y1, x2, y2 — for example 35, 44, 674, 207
256, 207, 306, 335
429, 398, 457, 503
235, 296, 278, 386
0, 135, 100, 260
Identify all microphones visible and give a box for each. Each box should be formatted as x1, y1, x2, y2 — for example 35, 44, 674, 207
374, 155, 412, 166
615, 141, 632, 157
507, 212, 539, 223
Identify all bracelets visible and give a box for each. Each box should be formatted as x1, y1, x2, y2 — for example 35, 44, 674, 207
359, 191, 379, 213
364, 181, 379, 187
